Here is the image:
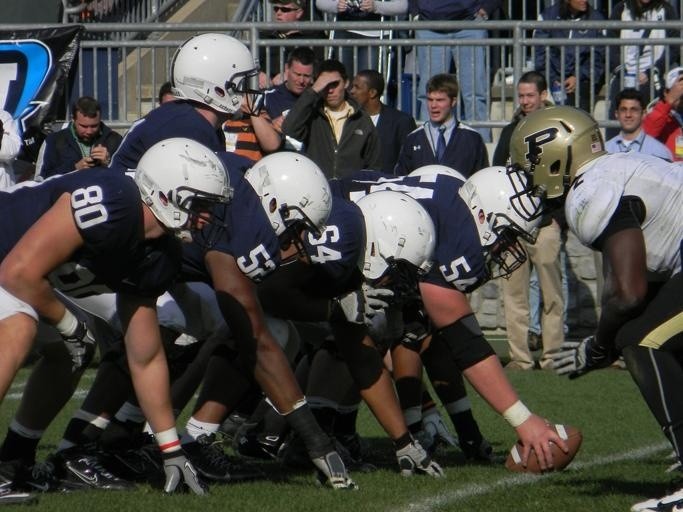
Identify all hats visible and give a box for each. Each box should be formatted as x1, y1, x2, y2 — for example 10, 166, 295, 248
269, 0, 306, 8
665, 67, 682, 89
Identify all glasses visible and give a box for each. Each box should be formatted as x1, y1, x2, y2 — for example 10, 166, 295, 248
615, 107, 642, 115
273, 6, 299, 13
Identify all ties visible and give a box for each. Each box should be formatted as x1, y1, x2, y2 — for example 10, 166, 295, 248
435, 128, 447, 164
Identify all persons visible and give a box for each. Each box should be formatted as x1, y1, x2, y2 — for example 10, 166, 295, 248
0, 0, 683, 512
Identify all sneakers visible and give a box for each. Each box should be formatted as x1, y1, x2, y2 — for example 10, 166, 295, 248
325, 424, 378, 474
630, 487, 683, 512
0, 474, 37, 506
181, 410, 379, 482
0, 430, 161, 498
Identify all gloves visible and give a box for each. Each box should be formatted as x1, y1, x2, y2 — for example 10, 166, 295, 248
395, 439, 447, 482
59, 320, 97, 374
333, 282, 395, 326
162, 448, 211, 496
304, 433, 361, 491
551, 335, 619, 379
422, 407, 463, 452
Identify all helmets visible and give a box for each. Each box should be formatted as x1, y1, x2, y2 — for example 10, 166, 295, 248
458, 166, 543, 279
168, 33, 265, 120
135, 137, 234, 250
510, 105, 608, 222
356, 190, 437, 308
244, 152, 333, 273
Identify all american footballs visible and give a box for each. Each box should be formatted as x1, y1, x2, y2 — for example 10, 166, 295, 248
504, 423, 583, 474
